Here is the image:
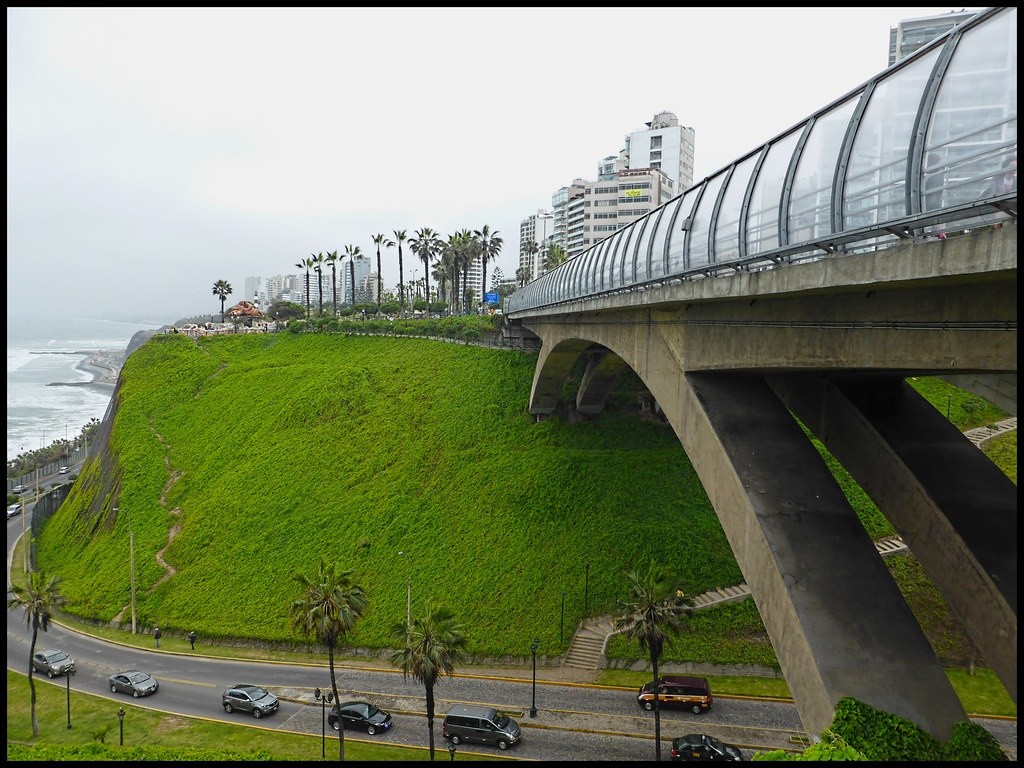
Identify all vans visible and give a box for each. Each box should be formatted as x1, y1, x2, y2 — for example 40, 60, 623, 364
59, 467, 70, 474
7, 505, 22, 516
443, 704, 522, 750
637, 676, 712, 714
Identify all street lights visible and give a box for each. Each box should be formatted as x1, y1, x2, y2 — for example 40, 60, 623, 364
561, 593, 564, 644
314, 687, 333, 757
585, 563, 590, 611
117, 709, 125, 746
530, 638, 541, 718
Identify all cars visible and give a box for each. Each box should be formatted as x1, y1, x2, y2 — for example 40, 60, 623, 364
13, 486, 28, 493
328, 702, 392, 735
222, 684, 279, 719
109, 670, 159, 698
32, 649, 74, 679
671, 734, 743, 762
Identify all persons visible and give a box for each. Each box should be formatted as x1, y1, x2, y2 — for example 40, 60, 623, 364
154, 627, 162, 648
189, 631, 197, 650
675, 588, 685, 599
